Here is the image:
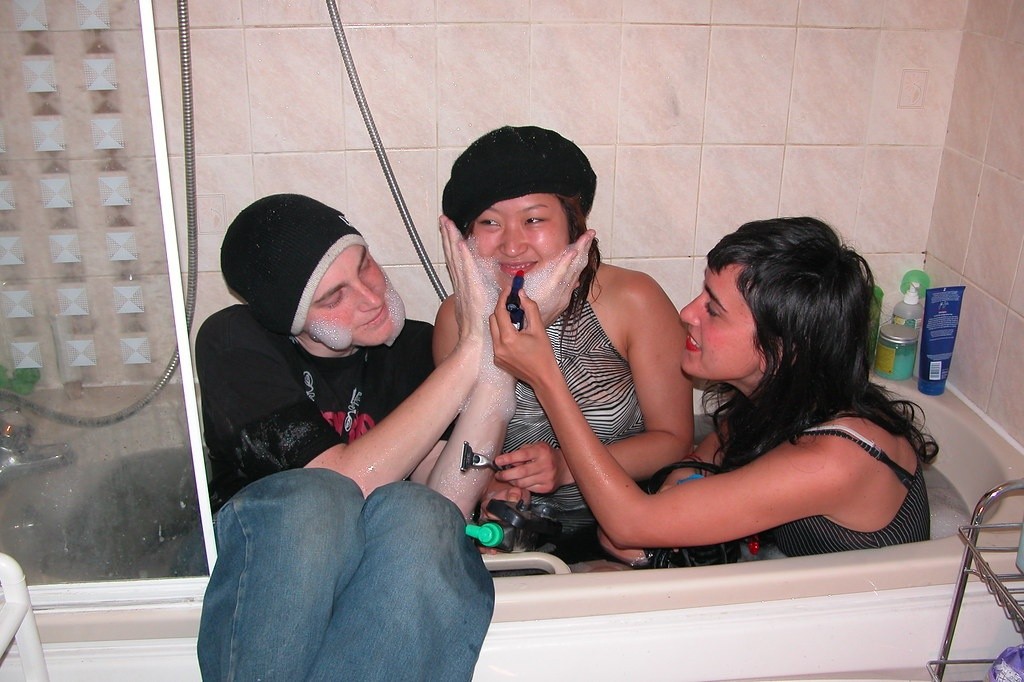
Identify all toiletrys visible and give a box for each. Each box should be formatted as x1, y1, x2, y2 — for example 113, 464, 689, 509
918, 285, 966, 396
866, 285, 885, 382
892, 281, 925, 328
873, 323, 923, 383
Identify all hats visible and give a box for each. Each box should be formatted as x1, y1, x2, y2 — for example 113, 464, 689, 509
441, 125, 598, 245
220, 193, 368, 338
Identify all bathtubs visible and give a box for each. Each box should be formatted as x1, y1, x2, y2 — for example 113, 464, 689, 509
0, 373, 1022, 682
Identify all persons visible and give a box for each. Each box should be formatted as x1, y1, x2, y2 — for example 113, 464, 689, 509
488, 215, 942, 569
194, 192, 599, 677
429, 124, 696, 557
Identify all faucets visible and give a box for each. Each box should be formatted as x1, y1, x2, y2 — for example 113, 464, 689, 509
0, 410, 77, 490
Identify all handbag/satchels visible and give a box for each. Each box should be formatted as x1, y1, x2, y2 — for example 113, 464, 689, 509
638, 459, 744, 570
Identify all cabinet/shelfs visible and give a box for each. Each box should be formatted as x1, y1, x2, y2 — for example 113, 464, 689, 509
925, 478, 1024, 682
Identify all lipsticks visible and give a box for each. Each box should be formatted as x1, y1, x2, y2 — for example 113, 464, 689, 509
506, 269, 525, 324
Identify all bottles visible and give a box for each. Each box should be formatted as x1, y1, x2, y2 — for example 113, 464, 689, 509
466, 522, 515, 551
